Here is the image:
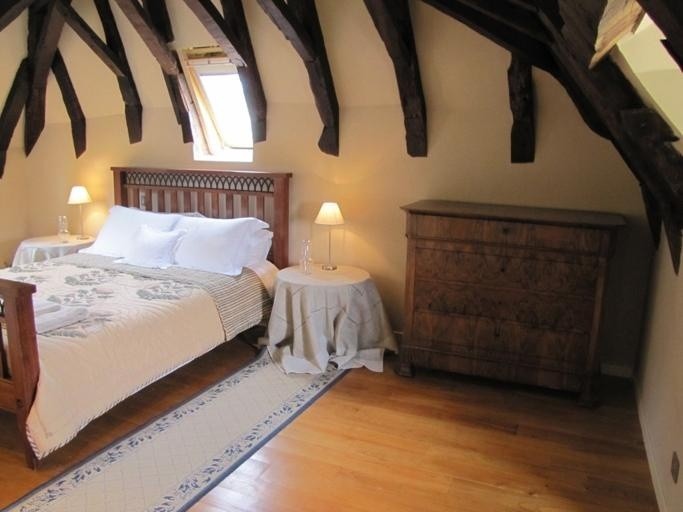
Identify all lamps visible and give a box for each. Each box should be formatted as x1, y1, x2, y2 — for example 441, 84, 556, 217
68, 186, 92, 240
314, 202, 345, 270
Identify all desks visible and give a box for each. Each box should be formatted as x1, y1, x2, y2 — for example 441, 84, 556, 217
258, 264, 397, 374
12, 235, 95, 267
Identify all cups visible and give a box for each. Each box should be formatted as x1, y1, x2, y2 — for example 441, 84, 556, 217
301, 240, 312, 274
58, 216, 70, 242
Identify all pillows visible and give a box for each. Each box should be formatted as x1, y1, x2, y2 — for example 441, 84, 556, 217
77, 205, 274, 275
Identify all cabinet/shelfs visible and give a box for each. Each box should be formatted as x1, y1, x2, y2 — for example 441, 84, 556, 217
399, 200, 628, 407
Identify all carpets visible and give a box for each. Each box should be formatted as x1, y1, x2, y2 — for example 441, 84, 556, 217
0, 356, 350, 512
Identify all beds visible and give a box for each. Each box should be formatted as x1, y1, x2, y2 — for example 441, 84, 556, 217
0, 167, 293, 469
